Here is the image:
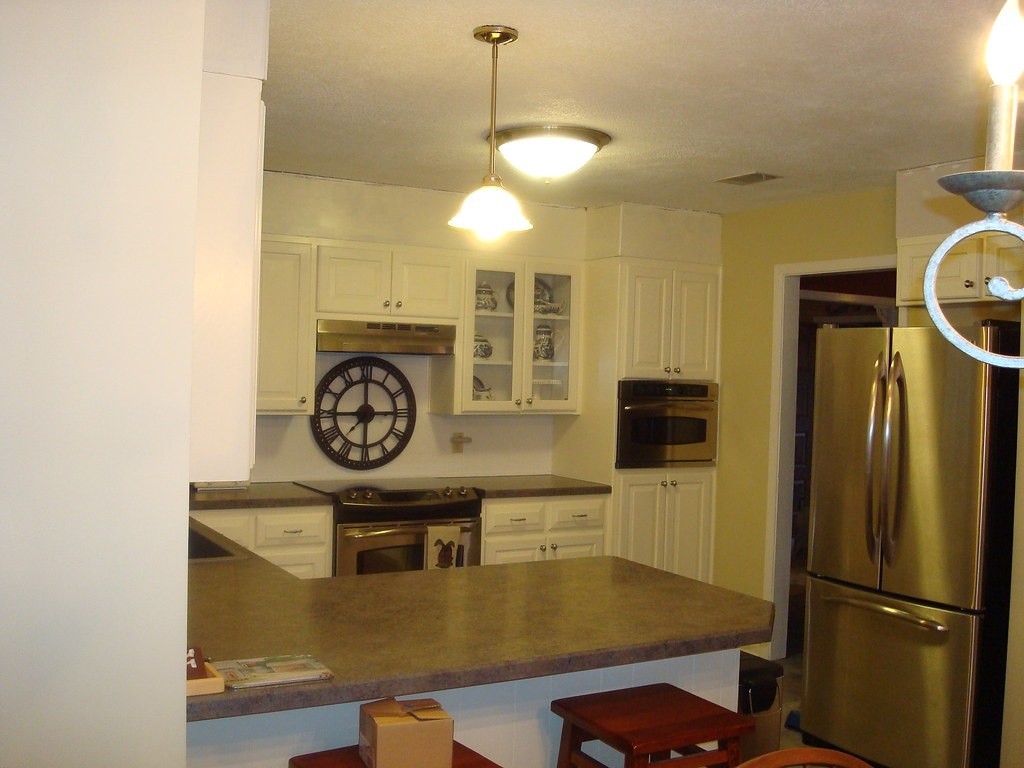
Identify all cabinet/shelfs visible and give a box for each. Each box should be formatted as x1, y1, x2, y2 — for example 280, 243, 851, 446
895, 228, 1024, 306
191, 506, 334, 577
614, 469, 714, 586
257, 172, 722, 416
479, 497, 606, 564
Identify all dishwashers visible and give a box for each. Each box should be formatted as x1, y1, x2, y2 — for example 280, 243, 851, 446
333, 518, 481, 576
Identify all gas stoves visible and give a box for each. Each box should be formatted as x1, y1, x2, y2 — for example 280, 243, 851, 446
292, 477, 479, 522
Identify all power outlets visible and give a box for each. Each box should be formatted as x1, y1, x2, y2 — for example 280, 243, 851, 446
452, 432, 464, 453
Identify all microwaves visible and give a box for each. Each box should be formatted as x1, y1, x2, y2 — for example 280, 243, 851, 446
614, 380, 719, 468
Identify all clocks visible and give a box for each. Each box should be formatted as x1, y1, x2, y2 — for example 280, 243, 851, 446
311, 355, 416, 470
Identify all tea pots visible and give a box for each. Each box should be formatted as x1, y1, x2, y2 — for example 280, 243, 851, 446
532, 321, 564, 363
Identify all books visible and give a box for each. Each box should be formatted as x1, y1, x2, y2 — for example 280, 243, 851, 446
193, 481, 250, 490
213, 654, 333, 687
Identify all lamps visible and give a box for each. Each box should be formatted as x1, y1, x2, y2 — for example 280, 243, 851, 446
487, 123, 609, 184
448, 25, 533, 245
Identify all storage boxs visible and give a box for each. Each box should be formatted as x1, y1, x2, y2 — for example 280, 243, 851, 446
358, 697, 453, 768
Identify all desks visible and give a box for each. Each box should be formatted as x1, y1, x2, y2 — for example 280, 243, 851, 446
287, 738, 505, 768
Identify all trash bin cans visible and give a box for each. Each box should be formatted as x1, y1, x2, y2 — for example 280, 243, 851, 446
733, 746, 873, 768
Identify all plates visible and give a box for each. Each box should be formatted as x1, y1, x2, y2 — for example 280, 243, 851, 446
473, 376, 487, 402
506, 277, 553, 312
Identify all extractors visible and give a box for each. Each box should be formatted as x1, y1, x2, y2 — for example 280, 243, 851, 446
317, 318, 456, 355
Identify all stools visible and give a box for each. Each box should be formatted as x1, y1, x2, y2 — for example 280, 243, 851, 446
552, 683, 757, 768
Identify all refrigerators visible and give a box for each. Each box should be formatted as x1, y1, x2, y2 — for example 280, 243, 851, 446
802, 317, 1021, 768
